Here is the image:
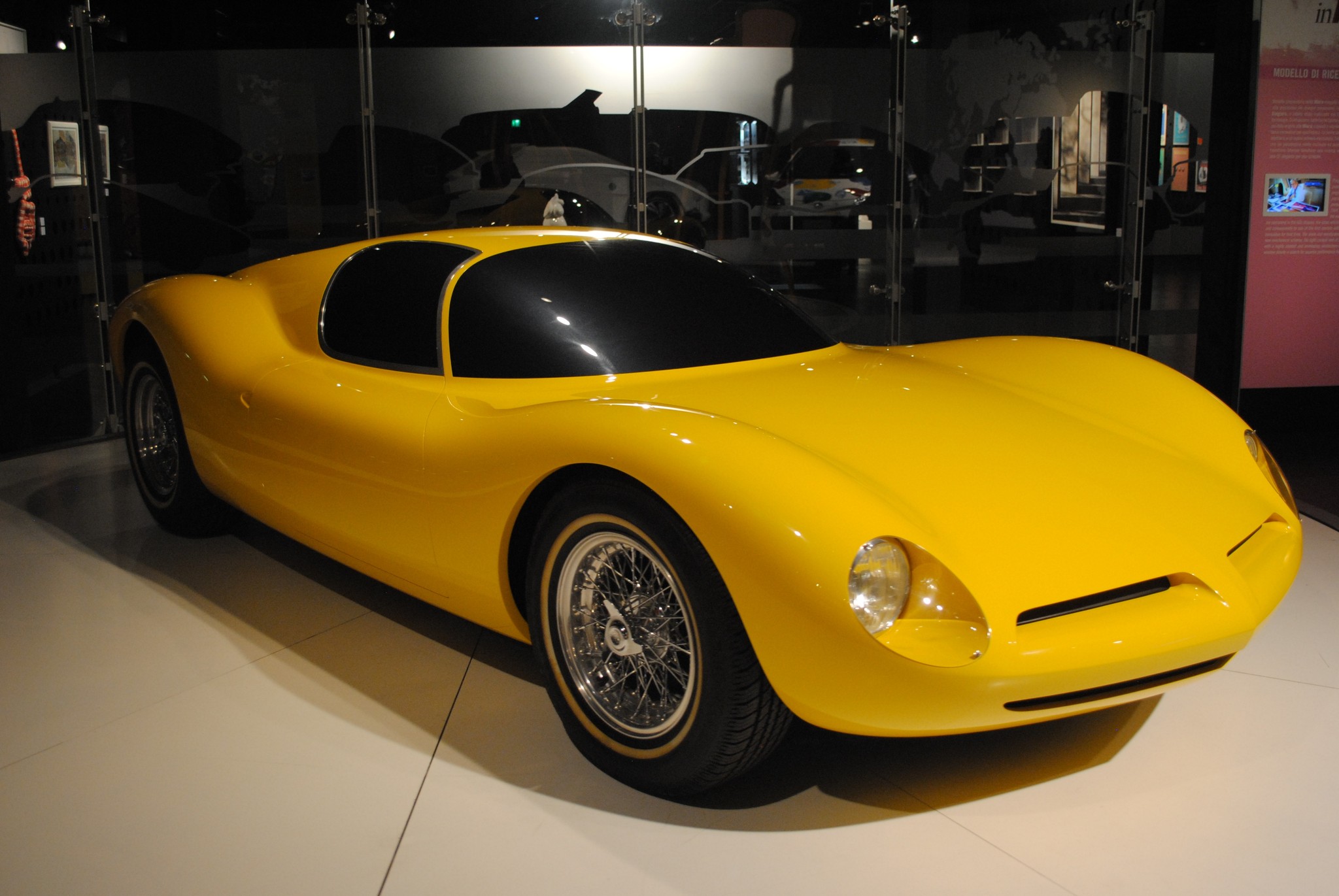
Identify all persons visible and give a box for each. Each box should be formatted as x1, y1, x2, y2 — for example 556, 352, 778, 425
1286, 179, 1305, 203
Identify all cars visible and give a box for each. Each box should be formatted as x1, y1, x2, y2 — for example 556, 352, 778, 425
766, 137, 878, 208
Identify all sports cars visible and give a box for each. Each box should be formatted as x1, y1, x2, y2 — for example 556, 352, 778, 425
105, 221, 1305, 804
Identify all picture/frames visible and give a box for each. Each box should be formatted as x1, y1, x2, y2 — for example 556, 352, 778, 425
47, 120, 81, 188
84, 125, 111, 187
